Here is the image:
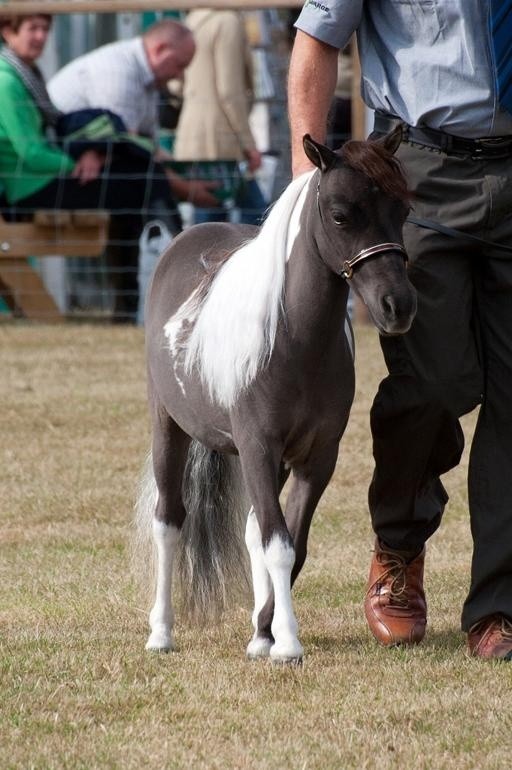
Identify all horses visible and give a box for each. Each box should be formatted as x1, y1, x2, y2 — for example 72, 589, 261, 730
126, 122, 421, 672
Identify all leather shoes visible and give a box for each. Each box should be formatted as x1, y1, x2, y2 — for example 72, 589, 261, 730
468, 614, 511, 664
364, 535, 427, 651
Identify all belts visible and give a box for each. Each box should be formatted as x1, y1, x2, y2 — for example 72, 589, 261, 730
372, 110, 511, 161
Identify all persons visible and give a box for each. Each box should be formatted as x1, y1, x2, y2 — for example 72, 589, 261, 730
43, 17, 226, 210
173, 5, 268, 231
281, 0, 512, 665
0, 12, 188, 325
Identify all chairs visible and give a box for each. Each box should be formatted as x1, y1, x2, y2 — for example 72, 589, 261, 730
0, 210, 109, 326
